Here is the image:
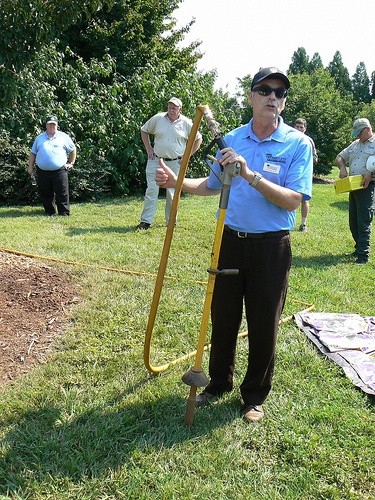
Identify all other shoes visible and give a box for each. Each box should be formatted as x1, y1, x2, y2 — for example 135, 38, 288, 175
299, 224, 306, 231
345, 251, 369, 265
136, 221, 151, 230
243, 402, 264, 421
195, 390, 219, 406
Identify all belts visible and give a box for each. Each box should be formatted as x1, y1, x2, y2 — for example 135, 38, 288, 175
153, 153, 182, 161
224, 225, 289, 238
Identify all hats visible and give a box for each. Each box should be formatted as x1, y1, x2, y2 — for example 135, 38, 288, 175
251, 66, 291, 91
352, 118, 370, 137
167, 97, 183, 109
46, 115, 58, 125
366, 156, 375, 173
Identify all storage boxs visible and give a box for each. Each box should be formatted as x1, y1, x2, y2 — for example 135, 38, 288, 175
333, 174, 364, 193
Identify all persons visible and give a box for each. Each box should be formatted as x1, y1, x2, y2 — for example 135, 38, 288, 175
28, 115, 76, 216
155, 66, 312, 421
336, 118, 375, 264
294, 117, 318, 231
136, 97, 202, 228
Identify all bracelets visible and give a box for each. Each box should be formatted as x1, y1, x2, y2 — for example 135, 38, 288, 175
248, 171, 262, 188
69, 162, 75, 165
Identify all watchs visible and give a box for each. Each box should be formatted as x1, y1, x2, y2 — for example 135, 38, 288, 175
370, 172, 375, 178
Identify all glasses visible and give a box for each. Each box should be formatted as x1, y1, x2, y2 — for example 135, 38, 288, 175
357, 127, 366, 137
252, 83, 288, 99
